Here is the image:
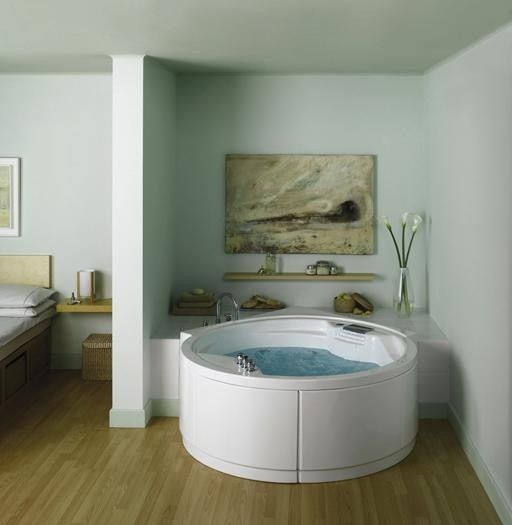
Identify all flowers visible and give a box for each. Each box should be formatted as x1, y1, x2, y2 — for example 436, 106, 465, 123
380, 211, 422, 315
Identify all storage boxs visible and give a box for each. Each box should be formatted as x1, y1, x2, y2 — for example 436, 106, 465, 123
82, 334, 112, 381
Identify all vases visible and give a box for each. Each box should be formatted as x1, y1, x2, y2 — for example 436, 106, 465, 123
391, 268, 414, 318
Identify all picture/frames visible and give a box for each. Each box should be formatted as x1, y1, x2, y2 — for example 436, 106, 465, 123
0, 157, 21, 237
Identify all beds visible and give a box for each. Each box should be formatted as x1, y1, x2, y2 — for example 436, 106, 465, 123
0, 256, 52, 409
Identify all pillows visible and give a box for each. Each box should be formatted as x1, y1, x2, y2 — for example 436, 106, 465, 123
0, 299, 56, 317
0, 285, 57, 307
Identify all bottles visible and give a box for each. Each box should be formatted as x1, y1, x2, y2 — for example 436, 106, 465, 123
264, 251, 276, 273
307, 262, 336, 275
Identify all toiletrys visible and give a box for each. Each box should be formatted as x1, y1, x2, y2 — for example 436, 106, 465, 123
305, 261, 337, 275
258, 251, 275, 276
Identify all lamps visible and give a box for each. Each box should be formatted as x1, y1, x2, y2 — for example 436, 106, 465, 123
76, 269, 96, 304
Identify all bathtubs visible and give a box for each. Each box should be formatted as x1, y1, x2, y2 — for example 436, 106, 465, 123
179, 314, 420, 484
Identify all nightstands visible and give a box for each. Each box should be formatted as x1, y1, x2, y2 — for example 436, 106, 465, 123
56, 298, 112, 312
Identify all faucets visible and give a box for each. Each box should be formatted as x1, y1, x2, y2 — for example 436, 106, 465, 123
216, 291, 239, 323
